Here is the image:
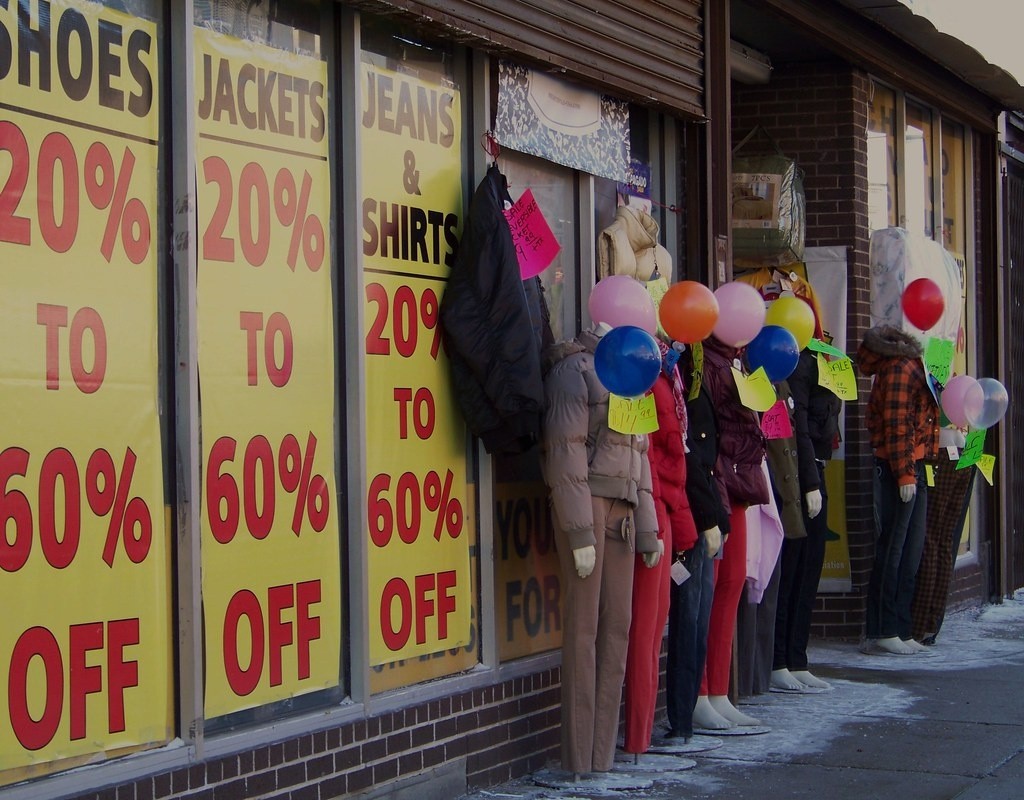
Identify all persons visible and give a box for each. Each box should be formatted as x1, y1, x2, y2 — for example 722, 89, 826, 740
669, 338, 732, 739
627, 338, 698, 753
739, 345, 845, 690
914, 423, 980, 647
694, 329, 770, 727
550, 321, 660, 776
861, 323, 931, 653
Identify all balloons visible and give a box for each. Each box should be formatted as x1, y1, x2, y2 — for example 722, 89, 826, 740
713, 282, 766, 349
904, 278, 946, 336
963, 378, 1010, 431
660, 281, 719, 346
941, 373, 984, 433
766, 295, 815, 351
746, 326, 799, 383
588, 275, 657, 337
596, 326, 662, 398
